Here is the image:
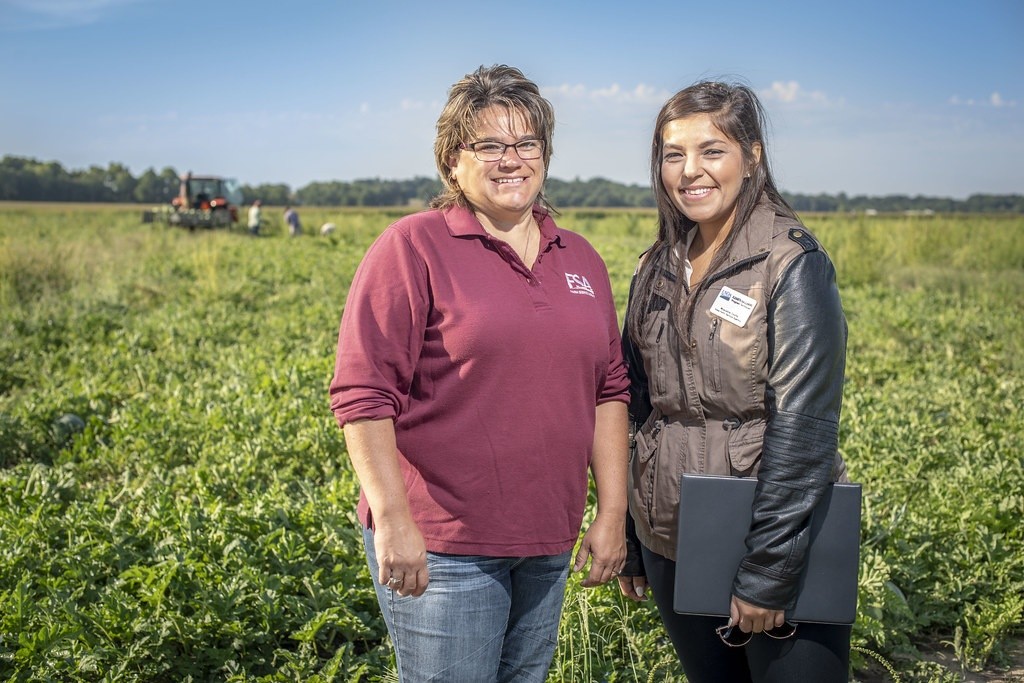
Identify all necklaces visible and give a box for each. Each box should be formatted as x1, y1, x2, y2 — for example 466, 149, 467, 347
475, 212, 533, 262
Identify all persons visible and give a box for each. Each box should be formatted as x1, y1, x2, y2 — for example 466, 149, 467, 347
247, 199, 261, 237
330, 64, 631, 683
617, 80, 853, 683
285, 204, 303, 239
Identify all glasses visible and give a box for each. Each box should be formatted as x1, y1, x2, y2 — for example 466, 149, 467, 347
456, 136, 547, 162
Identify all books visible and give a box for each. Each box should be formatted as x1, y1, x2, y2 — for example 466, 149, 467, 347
674, 472, 862, 625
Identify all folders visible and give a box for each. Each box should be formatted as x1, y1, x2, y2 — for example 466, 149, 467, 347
671, 476, 865, 628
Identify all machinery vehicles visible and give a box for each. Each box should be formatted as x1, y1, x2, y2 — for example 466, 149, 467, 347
141, 172, 242, 230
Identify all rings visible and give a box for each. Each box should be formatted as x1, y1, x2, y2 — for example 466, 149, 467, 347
613, 567, 622, 574
389, 577, 403, 584
